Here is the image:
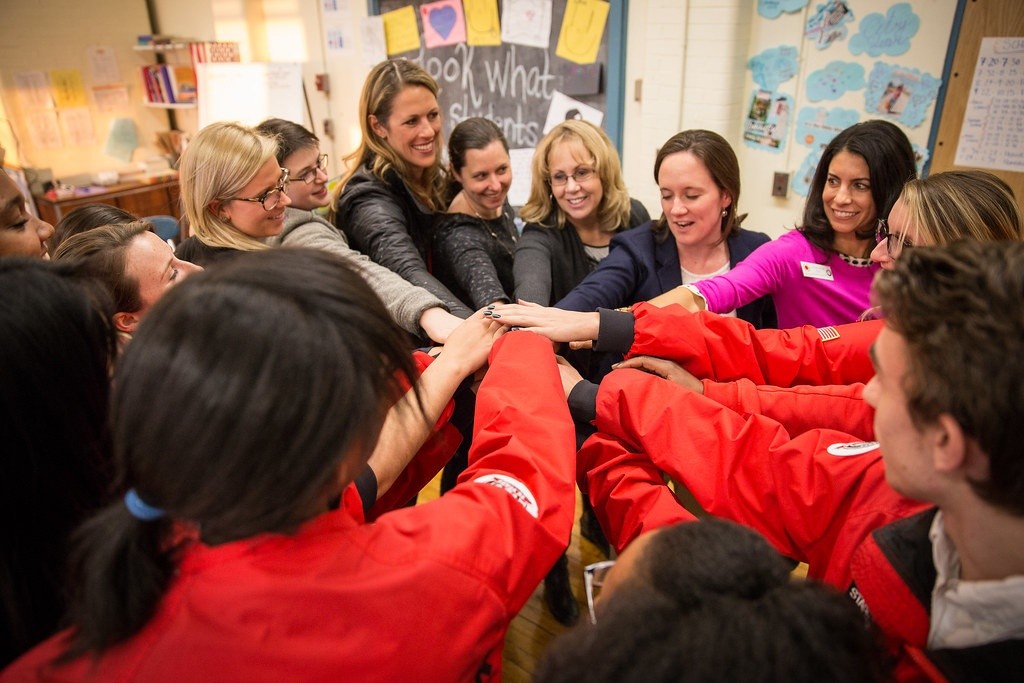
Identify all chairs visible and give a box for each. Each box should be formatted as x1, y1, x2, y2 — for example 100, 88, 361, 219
144, 216, 180, 251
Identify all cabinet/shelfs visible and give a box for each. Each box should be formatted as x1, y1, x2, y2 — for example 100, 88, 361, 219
34, 166, 190, 252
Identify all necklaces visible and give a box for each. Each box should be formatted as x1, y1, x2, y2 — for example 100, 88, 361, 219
463, 193, 517, 254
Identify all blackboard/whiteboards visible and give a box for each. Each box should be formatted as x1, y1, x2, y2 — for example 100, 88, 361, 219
367, 0, 629, 158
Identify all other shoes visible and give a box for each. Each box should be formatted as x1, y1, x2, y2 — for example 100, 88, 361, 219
580, 511, 611, 559
545, 564, 580, 627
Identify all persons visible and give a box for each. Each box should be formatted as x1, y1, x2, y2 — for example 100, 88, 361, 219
0, 58, 1024, 681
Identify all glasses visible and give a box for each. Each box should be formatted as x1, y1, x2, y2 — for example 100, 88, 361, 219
215, 167, 289, 211
876, 219, 933, 261
547, 165, 594, 186
289, 154, 328, 185
583, 559, 618, 626
